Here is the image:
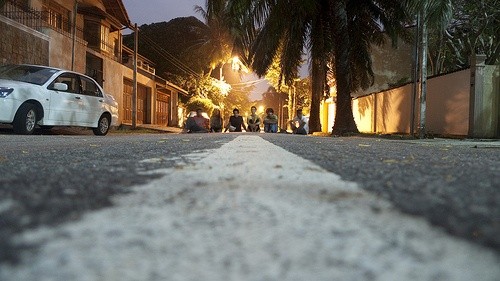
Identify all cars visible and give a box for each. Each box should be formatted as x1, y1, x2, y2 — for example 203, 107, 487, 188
0, 64, 120, 137
187, 112, 211, 129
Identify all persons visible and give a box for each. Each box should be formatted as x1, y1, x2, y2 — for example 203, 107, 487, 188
209, 108, 223, 132
247, 106, 261, 132
263, 108, 278, 133
224, 108, 247, 133
286, 108, 307, 135
188, 109, 206, 133
